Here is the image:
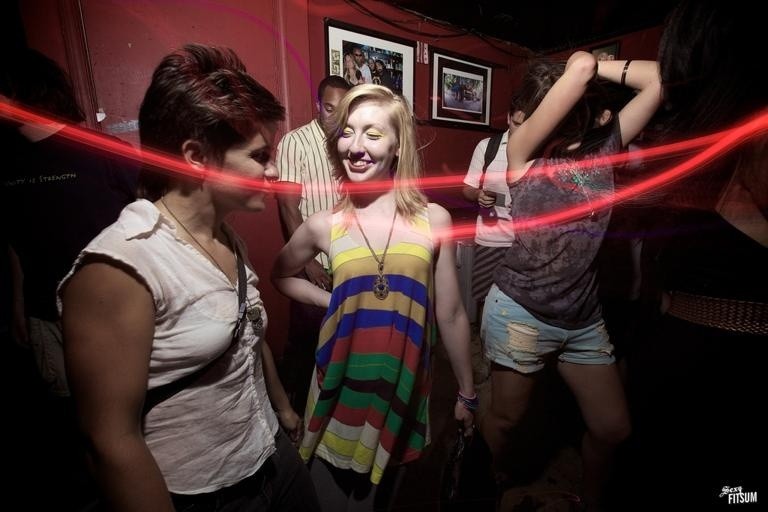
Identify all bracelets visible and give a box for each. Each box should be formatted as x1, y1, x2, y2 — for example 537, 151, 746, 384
457, 388, 481, 411
620, 59, 633, 87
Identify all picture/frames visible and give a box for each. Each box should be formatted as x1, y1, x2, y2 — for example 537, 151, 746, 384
428, 47, 495, 132
323, 16, 418, 122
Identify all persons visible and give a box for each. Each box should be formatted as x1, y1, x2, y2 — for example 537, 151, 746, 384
344, 46, 394, 89
460, 88, 530, 332
268, 82, 479, 512
0, 44, 162, 512
474, 51, 665, 511
658, 0, 768, 511
449, 74, 481, 103
54, 42, 321, 512
272, 75, 352, 415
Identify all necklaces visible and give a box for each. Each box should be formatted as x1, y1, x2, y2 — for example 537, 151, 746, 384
353, 199, 400, 301
160, 193, 261, 324
